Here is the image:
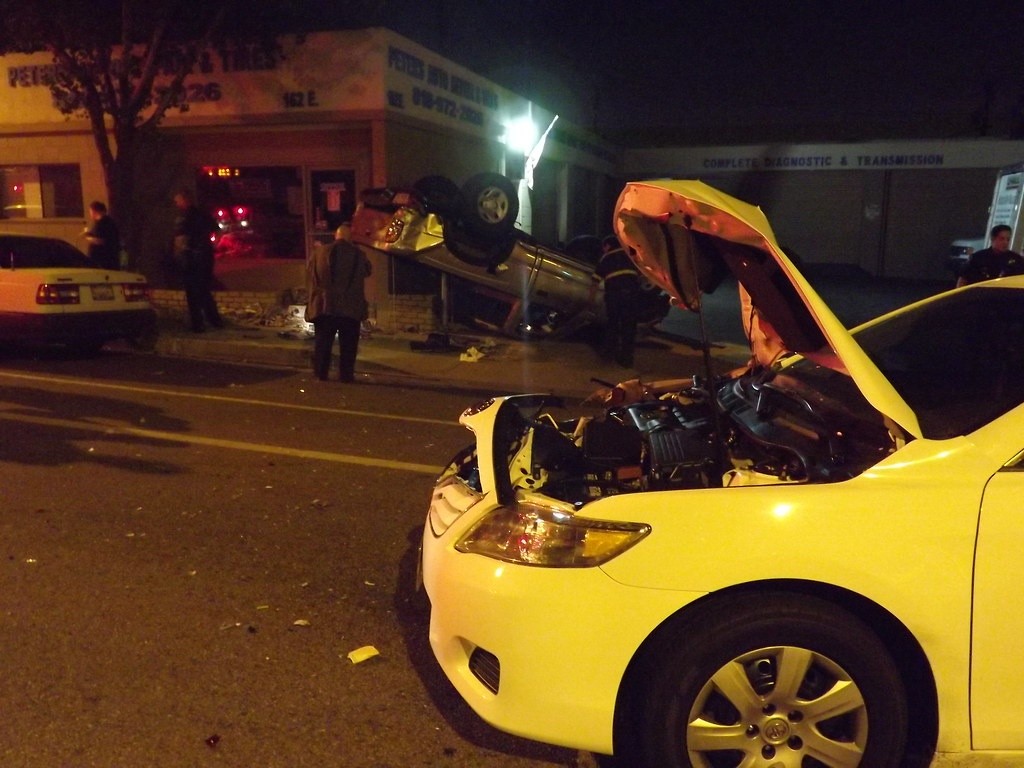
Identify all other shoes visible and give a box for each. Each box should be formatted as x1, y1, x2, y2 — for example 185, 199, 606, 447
339, 374, 356, 383
316, 371, 328, 381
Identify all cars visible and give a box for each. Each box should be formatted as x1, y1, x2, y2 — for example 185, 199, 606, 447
420, 178, 1024, 768
1, 233, 151, 361
349, 171, 671, 348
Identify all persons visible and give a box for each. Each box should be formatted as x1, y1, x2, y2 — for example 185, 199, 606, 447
171, 187, 227, 334
82, 200, 123, 271
304, 223, 374, 386
599, 232, 644, 370
954, 223, 1024, 297
